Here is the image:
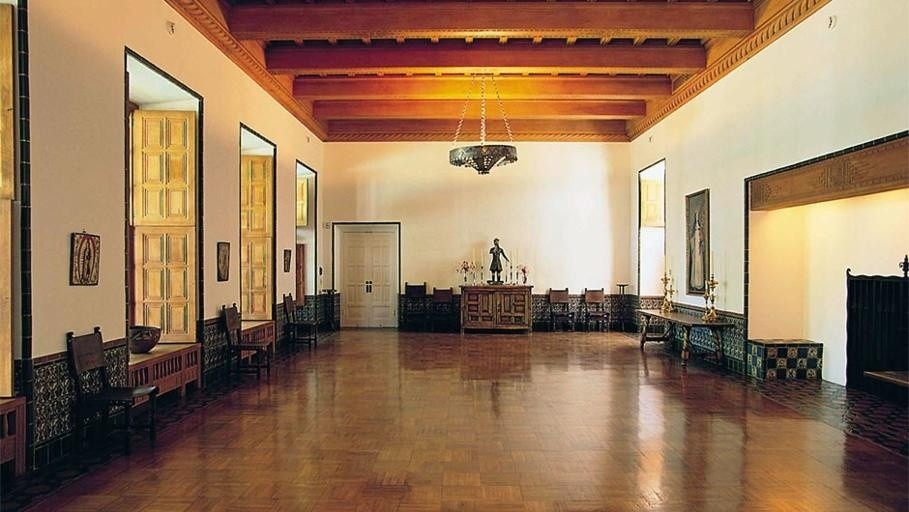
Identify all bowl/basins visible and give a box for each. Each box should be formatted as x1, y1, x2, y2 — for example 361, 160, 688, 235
128, 326, 161, 354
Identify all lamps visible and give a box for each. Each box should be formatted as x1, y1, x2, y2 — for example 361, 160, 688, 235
448, 74, 518, 176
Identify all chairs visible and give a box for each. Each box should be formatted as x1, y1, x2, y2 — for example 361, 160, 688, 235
583, 288, 610, 333
221, 303, 273, 384
65, 326, 159, 455
282, 292, 318, 351
548, 288, 575, 333
404, 281, 427, 331
432, 286, 454, 333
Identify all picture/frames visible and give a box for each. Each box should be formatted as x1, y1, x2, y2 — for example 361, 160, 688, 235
283, 249, 292, 272
216, 242, 230, 281
685, 188, 710, 295
70, 232, 100, 286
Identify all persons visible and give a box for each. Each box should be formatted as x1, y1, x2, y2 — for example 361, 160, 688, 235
690, 212, 704, 289
490, 239, 509, 282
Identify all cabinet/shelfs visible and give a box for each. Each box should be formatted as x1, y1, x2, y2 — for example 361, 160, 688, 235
1, 395, 27, 482
458, 284, 534, 335
127, 343, 200, 408
240, 319, 276, 366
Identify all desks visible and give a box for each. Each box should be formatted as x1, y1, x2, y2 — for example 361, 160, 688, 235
636, 309, 735, 368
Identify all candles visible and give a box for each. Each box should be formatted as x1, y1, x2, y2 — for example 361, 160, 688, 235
663, 253, 667, 273
670, 274, 674, 285
711, 249, 715, 275
705, 279, 709, 290
